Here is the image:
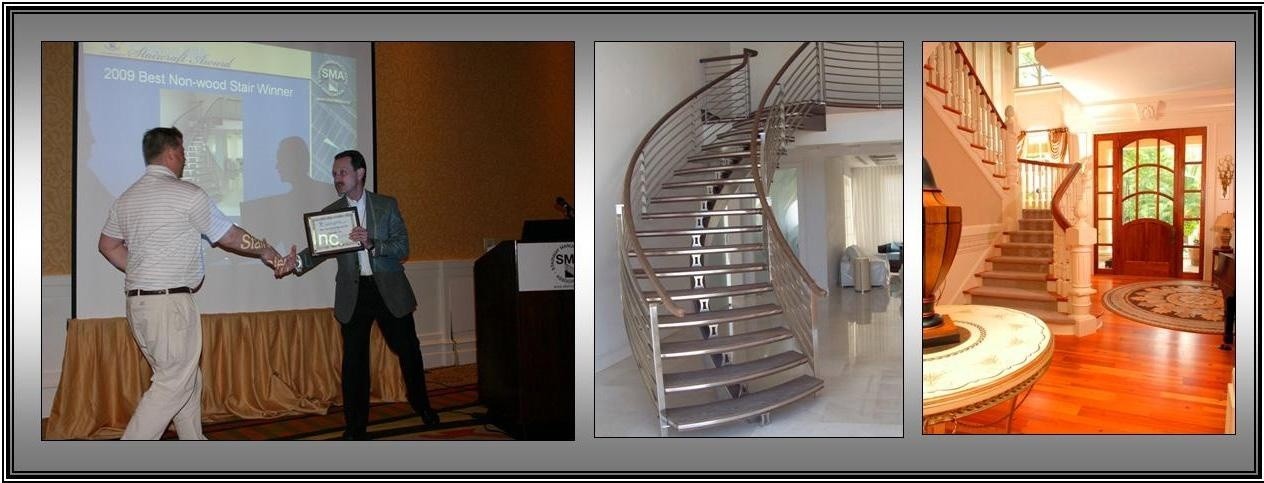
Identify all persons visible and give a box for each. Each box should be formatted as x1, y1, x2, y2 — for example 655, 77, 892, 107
97, 128, 283, 440
274, 150, 437, 440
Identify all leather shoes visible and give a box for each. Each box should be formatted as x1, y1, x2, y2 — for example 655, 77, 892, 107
422, 408, 440, 427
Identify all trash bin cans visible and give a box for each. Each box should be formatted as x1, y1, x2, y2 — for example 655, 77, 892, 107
855, 257, 871, 292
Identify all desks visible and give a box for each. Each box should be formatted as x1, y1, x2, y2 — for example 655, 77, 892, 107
1212, 252, 1235, 350
922, 303, 1055, 434
1211, 249, 1234, 286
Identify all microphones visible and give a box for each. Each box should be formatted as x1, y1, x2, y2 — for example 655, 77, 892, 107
556, 197, 573, 220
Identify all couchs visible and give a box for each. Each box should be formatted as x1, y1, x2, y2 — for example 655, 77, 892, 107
875, 241, 903, 260
840, 244, 891, 289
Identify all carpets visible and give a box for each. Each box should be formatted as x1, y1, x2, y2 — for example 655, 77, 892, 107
1101, 279, 1236, 335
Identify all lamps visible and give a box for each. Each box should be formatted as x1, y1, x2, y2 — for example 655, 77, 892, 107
1215, 214, 1235, 249
922, 187, 962, 347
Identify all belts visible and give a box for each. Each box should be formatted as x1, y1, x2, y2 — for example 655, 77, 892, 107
126, 288, 190, 296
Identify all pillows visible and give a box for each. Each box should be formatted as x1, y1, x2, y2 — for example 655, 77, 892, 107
878, 240, 900, 252
